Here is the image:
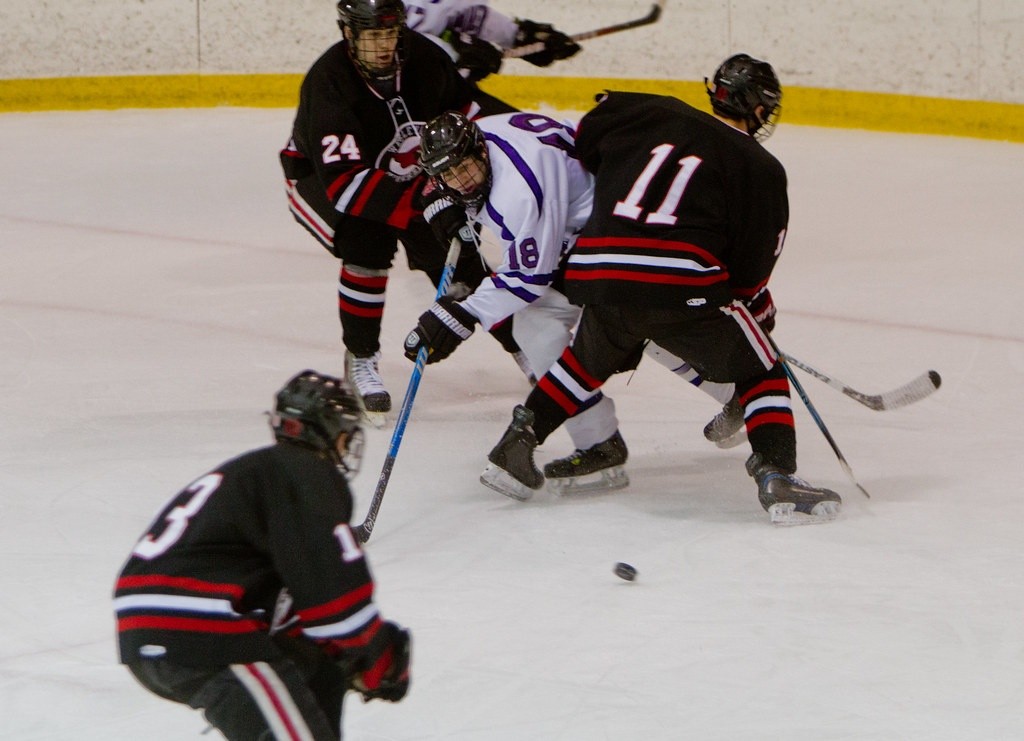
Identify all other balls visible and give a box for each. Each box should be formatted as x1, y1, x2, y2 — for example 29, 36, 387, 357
614, 562, 637, 583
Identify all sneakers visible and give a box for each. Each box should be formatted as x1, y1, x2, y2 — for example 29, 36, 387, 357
341, 342, 392, 428
541, 430, 629, 495
704, 391, 748, 449
511, 352, 539, 386
480, 403, 545, 501
745, 452, 841, 524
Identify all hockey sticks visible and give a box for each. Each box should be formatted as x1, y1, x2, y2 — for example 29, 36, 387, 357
766, 329, 874, 502
785, 352, 943, 413
501, 0, 668, 60
349, 237, 463, 546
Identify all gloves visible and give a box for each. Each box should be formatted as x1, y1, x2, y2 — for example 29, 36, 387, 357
345, 621, 412, 704
421, 191, 476, 259
743, 288, 777, 332
445, 32, 507, 82
512, 20, 582, 69
404, 301, 476, 365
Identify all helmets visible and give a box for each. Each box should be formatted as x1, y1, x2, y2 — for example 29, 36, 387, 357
704, 53, 782, 143
416, 114, 489, 207
338, 0, 405, 79
267, 370, 364, 479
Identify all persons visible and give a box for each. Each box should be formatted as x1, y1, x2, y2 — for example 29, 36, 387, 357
401, 0, 580, 83
114, 368, 410, 741
480, 53, 843, 527
402, 112, 749, 498
281, 0, 536, 428
